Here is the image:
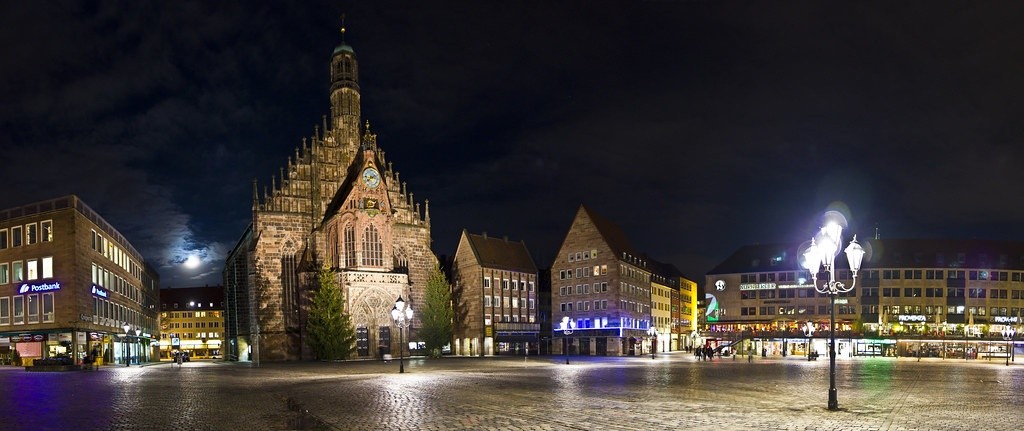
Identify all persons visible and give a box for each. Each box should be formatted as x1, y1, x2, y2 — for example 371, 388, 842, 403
696, 345, 714, 360
748, 349, 751, 363
733, 347, 737, 360
762, 348, 767, 357
909, 346, 912, 357
685, 345, 692, 354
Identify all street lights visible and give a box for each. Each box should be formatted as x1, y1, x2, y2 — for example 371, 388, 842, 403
647, 323, 659, 359
691, 329, 700, 356
559, 311, 576, 365
803, 320, 816, 362
1001, 322, 1014, 366
803, 219, 867, 411
391, 294, 415, 373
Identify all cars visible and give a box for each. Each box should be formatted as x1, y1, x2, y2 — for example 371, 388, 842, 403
174, 352, 191, 363
